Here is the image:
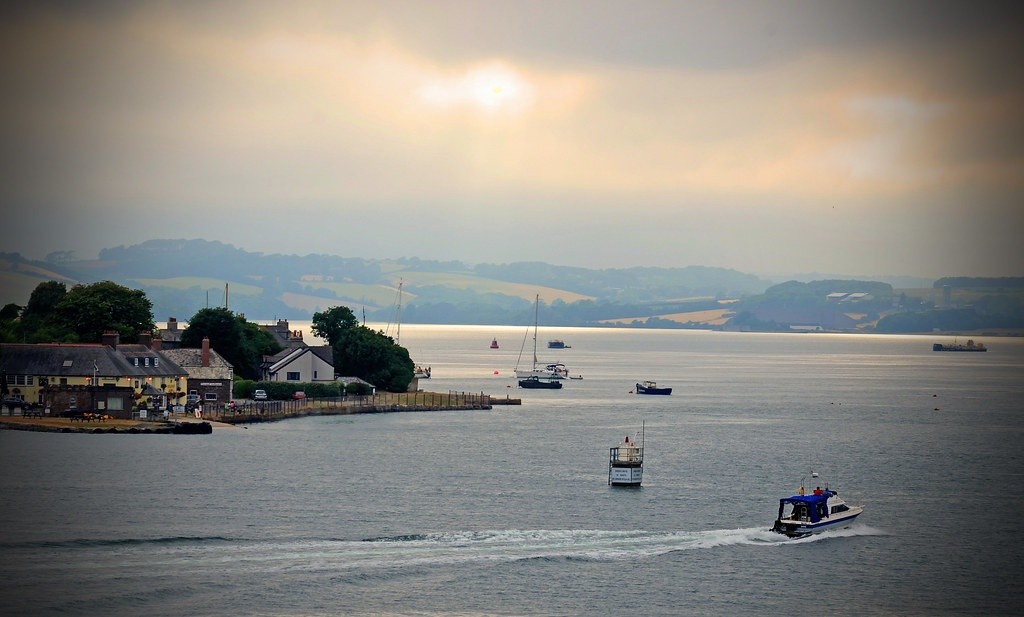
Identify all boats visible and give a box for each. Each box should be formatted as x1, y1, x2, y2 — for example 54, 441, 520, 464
518, 376, 562, 389
608, 421, 645, 488
547, 341, 571, 348
769, 454, 864, 539
933, 339, 987, 352
636, 381, 673, 395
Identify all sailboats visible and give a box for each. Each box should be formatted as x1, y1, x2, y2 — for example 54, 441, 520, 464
385, 277, 431, 379
514, 294, 583, 379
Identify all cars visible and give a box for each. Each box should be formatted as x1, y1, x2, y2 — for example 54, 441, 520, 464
292, 391, 307, 400
254, 390, 267, 401
